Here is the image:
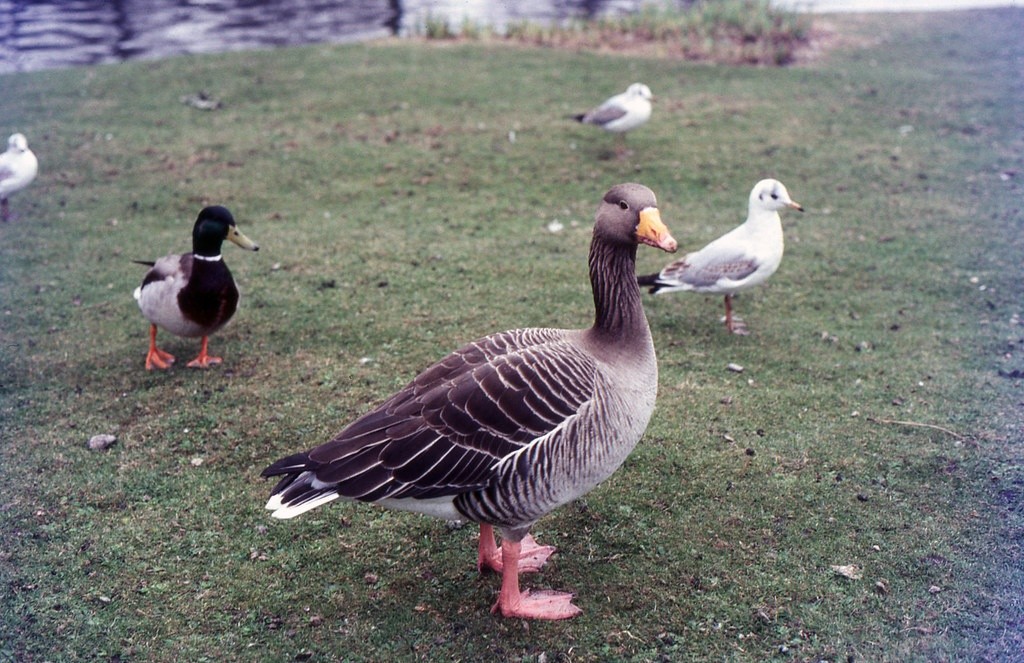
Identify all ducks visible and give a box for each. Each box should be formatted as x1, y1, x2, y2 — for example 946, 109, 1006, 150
259, 183, 679, 620
131, 205, 258, 371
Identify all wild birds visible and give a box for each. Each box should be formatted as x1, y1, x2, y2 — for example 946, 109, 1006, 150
562, 82, 657, 144
636, 178, 805, 335
0, 133, 39, 202
